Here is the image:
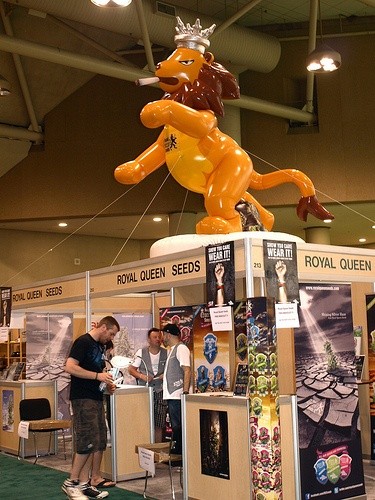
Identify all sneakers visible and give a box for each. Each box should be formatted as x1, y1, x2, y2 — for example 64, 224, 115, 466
78, 479, 109, 499
61, 477, 88, 500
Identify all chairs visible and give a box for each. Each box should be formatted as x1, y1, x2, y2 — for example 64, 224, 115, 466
17, 398, 71, 464
135, 425, 184, 500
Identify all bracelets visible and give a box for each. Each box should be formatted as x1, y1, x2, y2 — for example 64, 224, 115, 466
183, 392, 189, 394
96, 373, 98, 379
278, 279, 286, 287
217, 283, 224, 289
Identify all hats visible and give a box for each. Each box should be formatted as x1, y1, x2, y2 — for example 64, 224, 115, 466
160, 323, 180, 335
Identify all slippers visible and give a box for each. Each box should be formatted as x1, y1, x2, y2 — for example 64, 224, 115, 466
95, 478, 116, 487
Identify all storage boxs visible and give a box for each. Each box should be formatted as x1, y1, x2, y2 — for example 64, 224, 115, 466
29, 418, 69, 430
136, 441, 171, 456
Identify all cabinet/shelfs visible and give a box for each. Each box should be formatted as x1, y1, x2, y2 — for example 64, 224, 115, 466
0, 327, 28, 379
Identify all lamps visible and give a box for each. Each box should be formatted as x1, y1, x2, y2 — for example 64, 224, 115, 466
91, 0, 132, 7
0, 79, 11, 96
307, 0, 343, 72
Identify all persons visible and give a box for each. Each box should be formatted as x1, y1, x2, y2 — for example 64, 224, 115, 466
215, 263, 224, 304
160, 324, 190, 465
129, 328, 166, 443
275, 261, 288, 302
61, 316, 120, 500
